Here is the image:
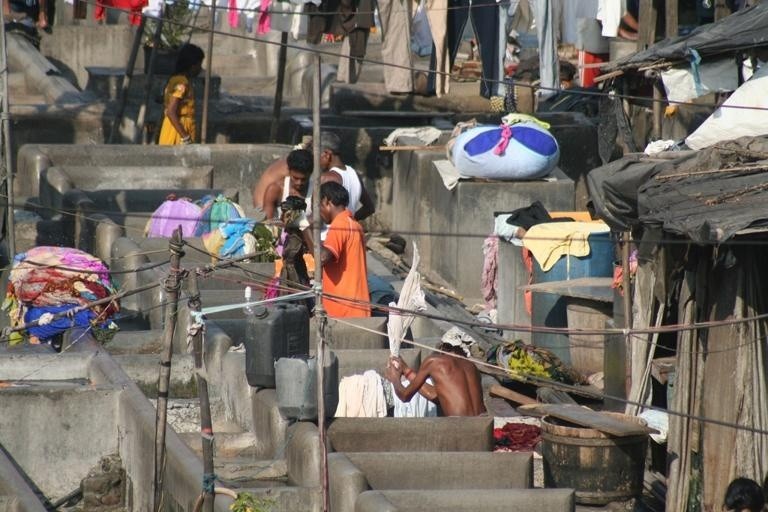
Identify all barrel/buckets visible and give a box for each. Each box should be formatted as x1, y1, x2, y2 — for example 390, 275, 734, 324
528, 230, 615, 369
540, 413, 647, 504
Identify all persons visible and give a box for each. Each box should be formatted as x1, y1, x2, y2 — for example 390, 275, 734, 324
2, 0, 49, 29
384, 342, 487, 417
722, 478, 766, 512
252, 130, 375, 319
158, 43, 206, 146
596, 0, 641, 41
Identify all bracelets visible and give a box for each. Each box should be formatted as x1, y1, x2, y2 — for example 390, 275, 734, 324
181, 134, 192, 144
